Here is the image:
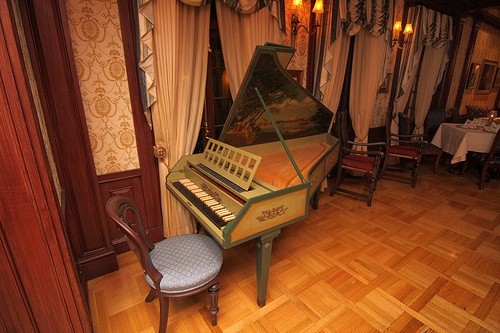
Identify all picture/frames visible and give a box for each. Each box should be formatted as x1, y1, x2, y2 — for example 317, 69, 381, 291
287, 70, 304, 86
466, 60, 500, 96
378, 73, 392, 93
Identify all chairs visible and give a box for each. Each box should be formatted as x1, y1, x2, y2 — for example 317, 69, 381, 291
106, 195, 223, 333
406, 110, 442, 175
462, 128, 500, 190
328, 109, 390, 207
377, 111, 428, 188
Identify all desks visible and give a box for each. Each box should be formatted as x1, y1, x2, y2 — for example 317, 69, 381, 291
430, 117, 500, 177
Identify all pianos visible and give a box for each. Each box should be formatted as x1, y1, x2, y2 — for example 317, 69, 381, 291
164, 41, 342, 310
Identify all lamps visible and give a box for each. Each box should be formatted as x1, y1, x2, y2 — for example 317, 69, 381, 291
289, 0, 325, 36
390, 21, 413, 49
